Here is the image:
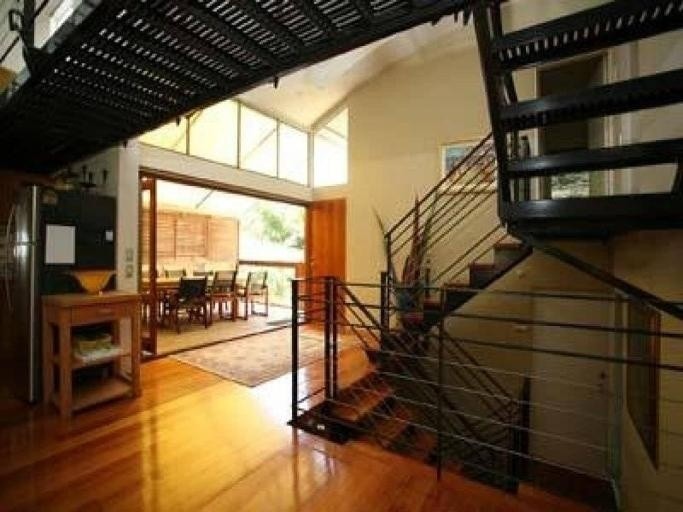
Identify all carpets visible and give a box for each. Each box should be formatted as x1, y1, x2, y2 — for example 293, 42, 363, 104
166, 328, 360, 388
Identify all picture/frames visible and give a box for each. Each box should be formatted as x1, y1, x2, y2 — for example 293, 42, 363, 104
439, 138, 497, 195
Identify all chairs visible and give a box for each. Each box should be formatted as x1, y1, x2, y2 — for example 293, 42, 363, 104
142, 267, 269, 333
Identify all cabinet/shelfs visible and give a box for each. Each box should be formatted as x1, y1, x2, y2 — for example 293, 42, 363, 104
141, 210, 207, 265
207, 217, 240, 263
41, 288, 143, 420
